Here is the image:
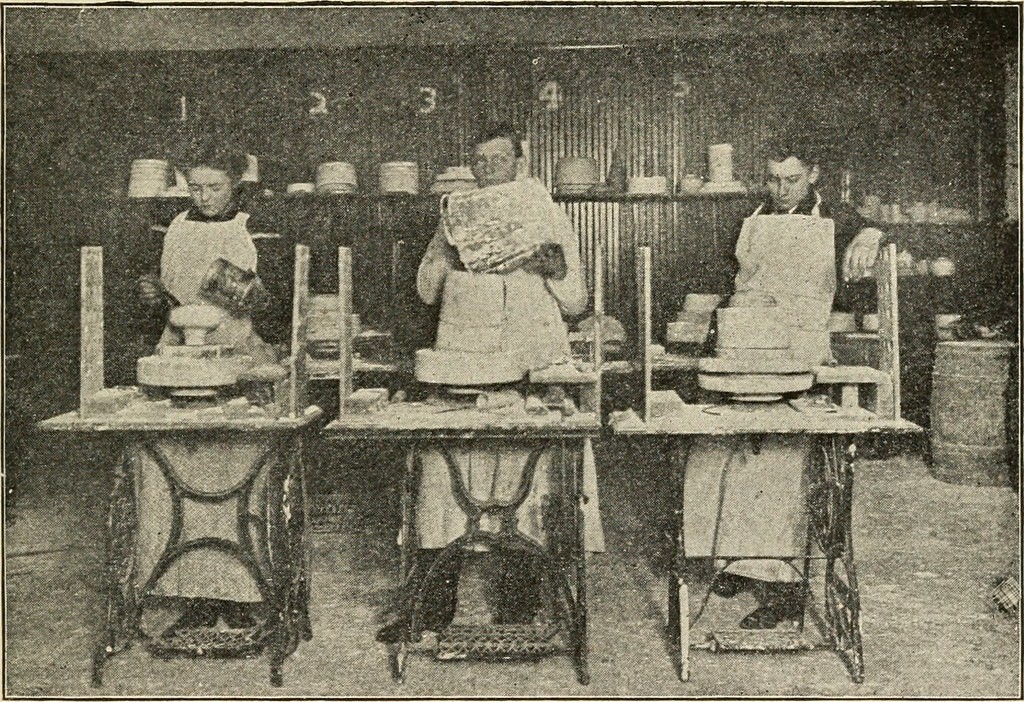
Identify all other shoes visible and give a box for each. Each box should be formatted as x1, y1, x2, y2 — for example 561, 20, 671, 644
713, 583, 737, 598
161, 610, 217, 643
740, 608, 784, 630
378, 618, 448, 643
221, 611, 256, 628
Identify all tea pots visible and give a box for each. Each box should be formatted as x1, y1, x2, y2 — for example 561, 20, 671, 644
680, 172, 703, 193
898, 248, 957, 277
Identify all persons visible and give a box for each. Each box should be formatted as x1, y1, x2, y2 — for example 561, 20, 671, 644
682, 137, 888, 633
135, 148, 293, 639
372, 123, 604, 643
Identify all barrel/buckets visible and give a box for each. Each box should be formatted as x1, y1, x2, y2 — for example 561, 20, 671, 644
929, 340, 1018, 487
201, 258, 254, 307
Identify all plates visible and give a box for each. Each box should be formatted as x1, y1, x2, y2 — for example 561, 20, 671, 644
430, 167, 479, 194
285, 183, 313, 193
703, 141, 750, 194
860, 313, 880, 330
556, 158, 604, 195
317, 160, 359, 193
126, 157, 169, 199
663, 293, 723, 341
624, 176, 672, 195
308, 295, 342, 357
827, 312, 857, 332
377, 160, 420, 196
